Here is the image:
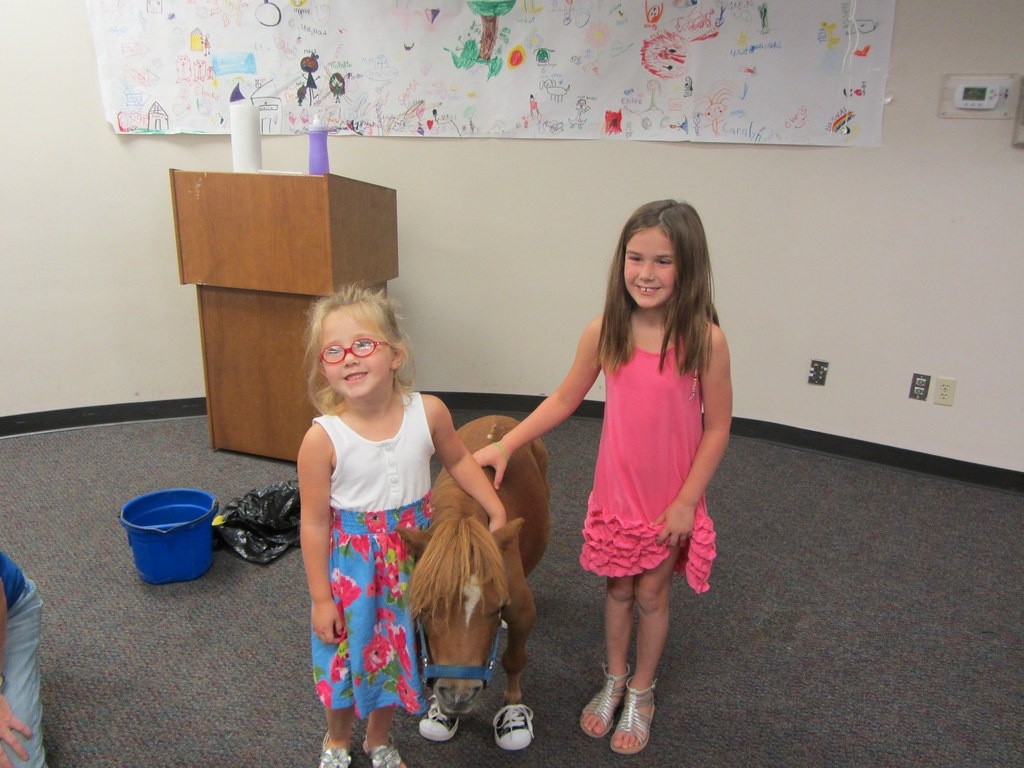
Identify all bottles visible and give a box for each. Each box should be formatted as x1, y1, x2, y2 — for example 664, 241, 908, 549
307, 114, 329, 176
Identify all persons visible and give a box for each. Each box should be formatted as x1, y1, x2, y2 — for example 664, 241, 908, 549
0, 551, 48, 768
297, 287, 506, 768
474, 200, 732, 753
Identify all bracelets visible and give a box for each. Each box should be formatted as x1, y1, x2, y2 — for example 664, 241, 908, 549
493, 442, 509, 462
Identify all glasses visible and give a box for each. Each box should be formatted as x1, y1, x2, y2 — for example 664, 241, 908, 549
319, 338, 389, 364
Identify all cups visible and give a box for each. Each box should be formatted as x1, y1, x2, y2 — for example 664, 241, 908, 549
229, 105, 263, 173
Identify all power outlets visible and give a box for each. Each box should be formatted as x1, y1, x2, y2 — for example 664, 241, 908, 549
810, 360, 828, 385
933, 377, 958, 405
910, 372, 931, 400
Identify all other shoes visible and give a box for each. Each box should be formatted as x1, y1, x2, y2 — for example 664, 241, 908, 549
418, 694, 459, 742
492, 703, 535, 751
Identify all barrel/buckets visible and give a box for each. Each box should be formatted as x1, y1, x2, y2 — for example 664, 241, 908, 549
116, 488, 219, 584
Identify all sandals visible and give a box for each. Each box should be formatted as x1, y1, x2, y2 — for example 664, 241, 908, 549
579, 662, 630, 739
318, 730, 351, 768
362, 733, 407, 768
610, 675, 655, 755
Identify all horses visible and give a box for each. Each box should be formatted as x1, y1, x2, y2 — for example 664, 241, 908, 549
395, 415, 551, 750
